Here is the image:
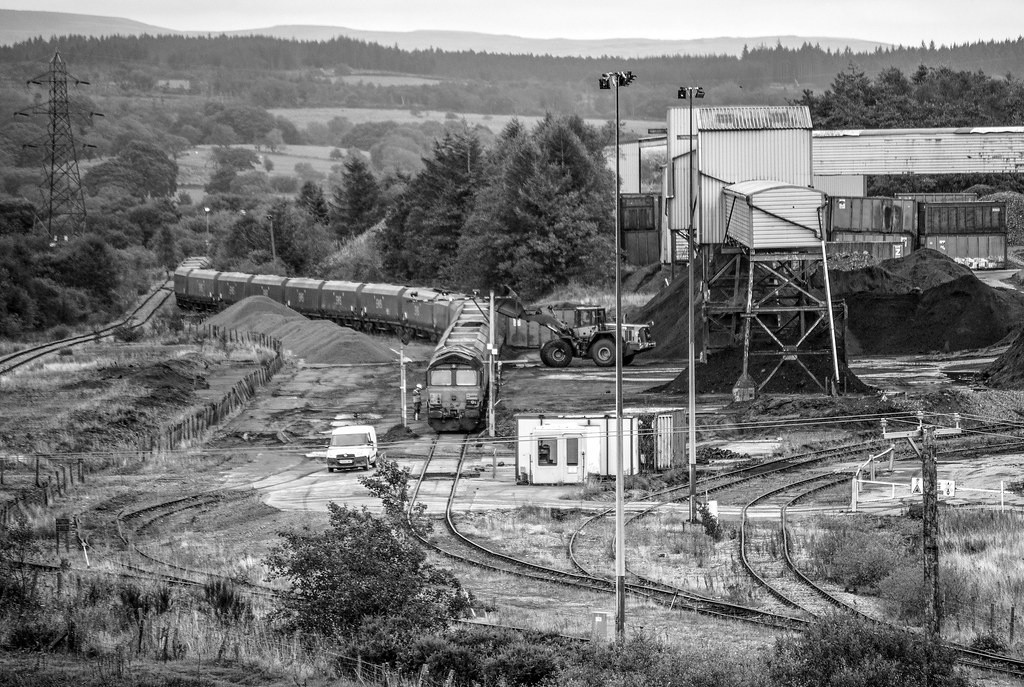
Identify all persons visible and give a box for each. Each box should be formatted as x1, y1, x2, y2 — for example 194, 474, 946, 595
583, 311, 595, 327
412, 384, 422, 421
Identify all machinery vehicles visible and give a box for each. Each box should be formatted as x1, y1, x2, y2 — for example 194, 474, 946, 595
487, 284, 657, 368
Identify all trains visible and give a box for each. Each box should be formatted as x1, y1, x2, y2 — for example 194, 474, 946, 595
173, 254, 499, 434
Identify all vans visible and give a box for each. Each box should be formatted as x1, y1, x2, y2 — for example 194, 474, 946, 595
325, 425, 379, 473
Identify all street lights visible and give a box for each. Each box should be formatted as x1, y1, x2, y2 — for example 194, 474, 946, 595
599, 69, 640, 650
203, 205, 210, 245
676, 83, 708, 530
265, 215, 278, 266
